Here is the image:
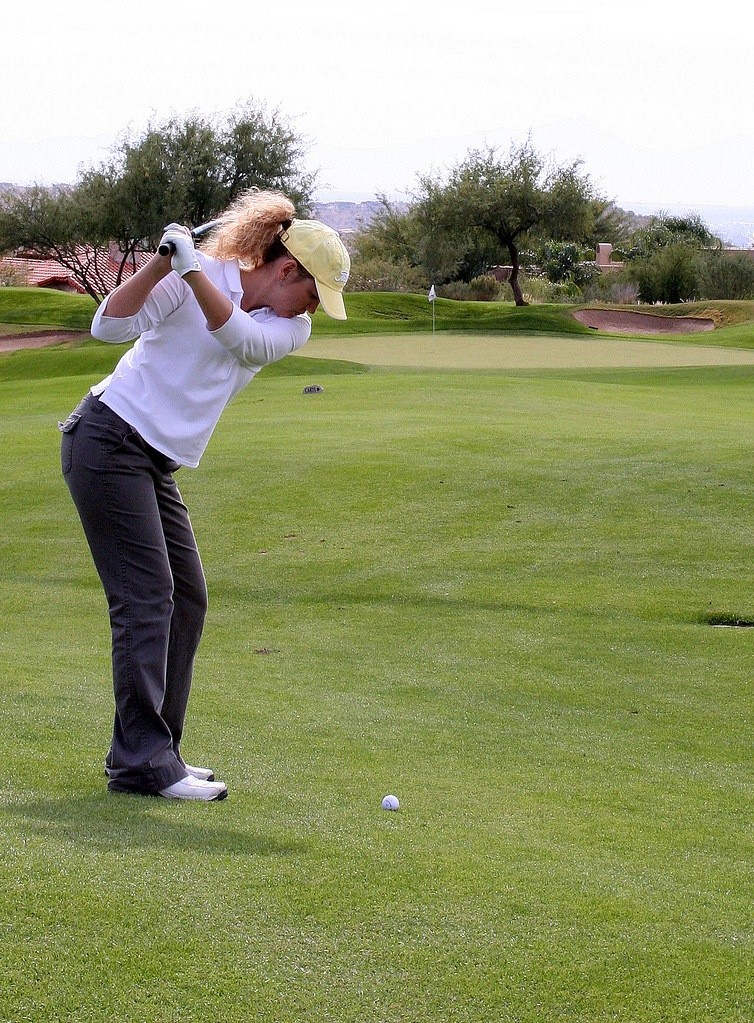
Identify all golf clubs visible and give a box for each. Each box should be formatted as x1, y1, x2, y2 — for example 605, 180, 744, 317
156, 217, 224, 259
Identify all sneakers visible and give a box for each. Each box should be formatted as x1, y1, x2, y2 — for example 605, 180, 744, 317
104, 756, 215, 782
107, 772, 228, 802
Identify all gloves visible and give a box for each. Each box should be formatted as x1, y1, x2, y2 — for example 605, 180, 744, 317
158, 223, 202, 277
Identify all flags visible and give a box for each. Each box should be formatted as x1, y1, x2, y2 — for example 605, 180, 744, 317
428, 285, 436, 301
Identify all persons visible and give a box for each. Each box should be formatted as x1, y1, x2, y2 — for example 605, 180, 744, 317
58, 189, 352, 802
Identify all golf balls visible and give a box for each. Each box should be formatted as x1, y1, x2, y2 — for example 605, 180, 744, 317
382, 794, 399, 811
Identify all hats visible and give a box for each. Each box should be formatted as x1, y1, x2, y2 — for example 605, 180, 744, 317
277, 218, 351, 321
